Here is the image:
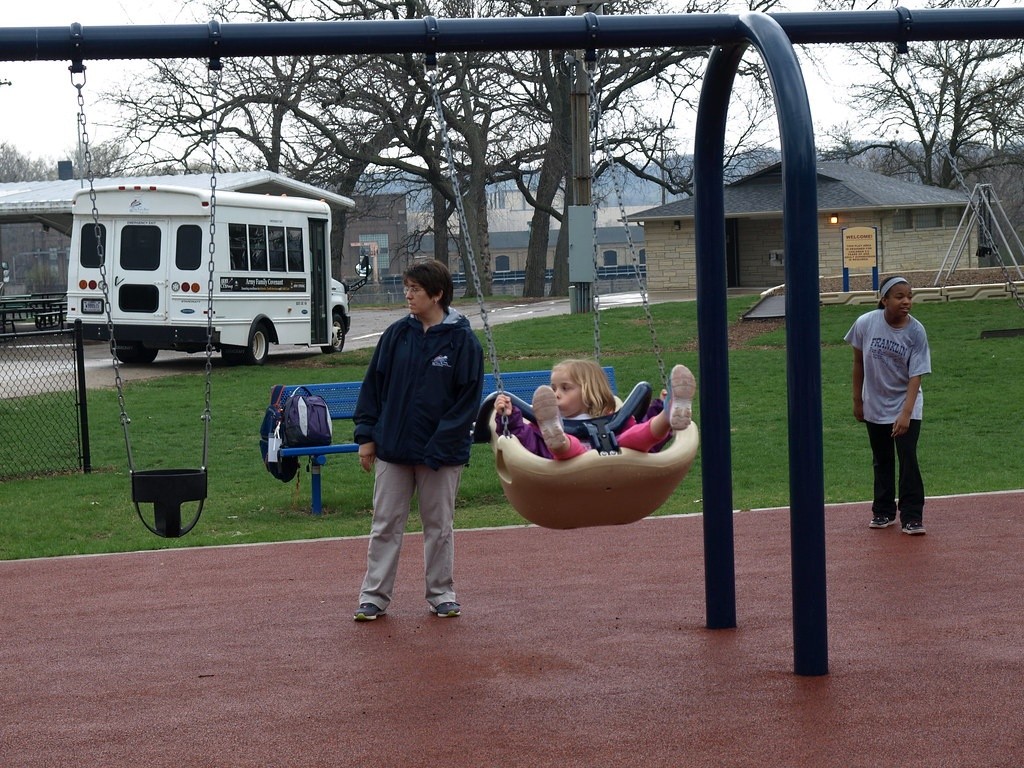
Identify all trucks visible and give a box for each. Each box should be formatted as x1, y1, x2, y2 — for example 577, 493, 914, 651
66, 185, 373, 365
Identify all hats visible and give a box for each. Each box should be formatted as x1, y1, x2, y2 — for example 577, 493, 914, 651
877, 275, 908, 310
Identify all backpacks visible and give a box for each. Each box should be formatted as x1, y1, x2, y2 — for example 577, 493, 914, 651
283, 386, 332, 447
259, 386, 300, 482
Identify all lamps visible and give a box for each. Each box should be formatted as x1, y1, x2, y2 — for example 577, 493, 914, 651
831, 213, 839, 225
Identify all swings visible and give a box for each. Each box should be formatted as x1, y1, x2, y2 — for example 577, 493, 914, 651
66, 15, 223, 540
423, 11, 704, 534
893, 18, 1024, 312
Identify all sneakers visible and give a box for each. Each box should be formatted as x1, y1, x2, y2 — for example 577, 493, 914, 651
429, 602, 461, 618
662, 364, 695, 430
902, 521, 925, 535
868, 514, 896, 528
531, 385, 566, 452
354, 603, 385, 621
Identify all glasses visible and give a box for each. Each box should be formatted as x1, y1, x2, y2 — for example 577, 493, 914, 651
403, 286, 427, 295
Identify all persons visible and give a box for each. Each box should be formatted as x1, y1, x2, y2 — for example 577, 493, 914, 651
354, 260, 485, 620
844, 276, 931, 534
494, 361, 696, 460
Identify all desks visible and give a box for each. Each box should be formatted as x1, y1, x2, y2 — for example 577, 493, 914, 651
0, 292, 66, 341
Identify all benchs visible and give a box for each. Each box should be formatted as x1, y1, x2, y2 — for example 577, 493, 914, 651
34, 311, 67, 316
270, 366, 616, 516
0, 306, 22, 308
0, 319, 26, 322
